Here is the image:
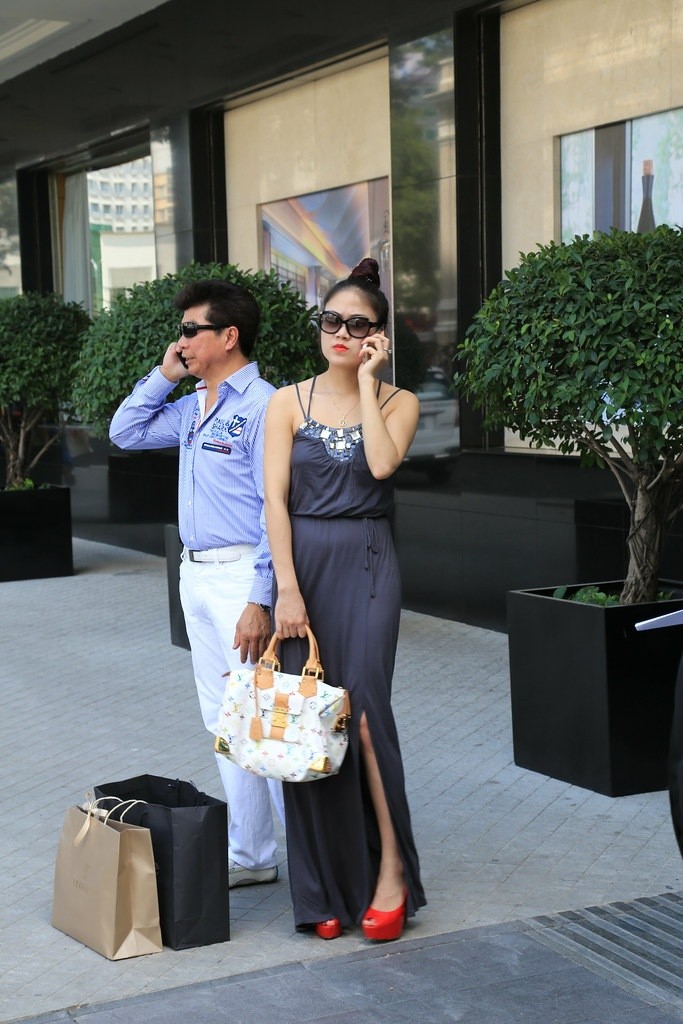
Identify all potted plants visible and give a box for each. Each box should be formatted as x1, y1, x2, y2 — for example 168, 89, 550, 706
449, 223, 683, 800
0, 262, 324, 650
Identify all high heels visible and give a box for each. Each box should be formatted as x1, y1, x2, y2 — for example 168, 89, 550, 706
361, 884, 409, 940
314, 918, 341, 939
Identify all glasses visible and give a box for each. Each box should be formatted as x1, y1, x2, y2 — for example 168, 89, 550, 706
177, 322, 222, 339
318, 311, 384, 339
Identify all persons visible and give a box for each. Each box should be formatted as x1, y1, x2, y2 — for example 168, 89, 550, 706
263, 257, 428, 941
109, 279, 285, 890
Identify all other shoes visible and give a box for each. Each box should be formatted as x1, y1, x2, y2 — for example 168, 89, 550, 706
227, 858, 279, 889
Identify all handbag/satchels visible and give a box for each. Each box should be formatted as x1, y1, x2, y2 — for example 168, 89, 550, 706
49, 801, 163, 961
215, 621, 352, 783
93, 774, 230, 951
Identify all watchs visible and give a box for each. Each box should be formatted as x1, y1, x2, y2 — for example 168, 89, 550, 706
254, 602, 271, 611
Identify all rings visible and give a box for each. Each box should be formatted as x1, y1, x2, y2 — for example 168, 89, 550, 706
384, 348, 391, 354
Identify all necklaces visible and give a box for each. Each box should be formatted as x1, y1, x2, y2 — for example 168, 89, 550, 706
323, 374, 360, 427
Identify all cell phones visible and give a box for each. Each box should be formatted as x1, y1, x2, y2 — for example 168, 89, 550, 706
363, 325, 387, 363
176, 352, 188, 369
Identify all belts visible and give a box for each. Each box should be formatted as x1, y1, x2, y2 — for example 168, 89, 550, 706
179, 545, 256, 563
